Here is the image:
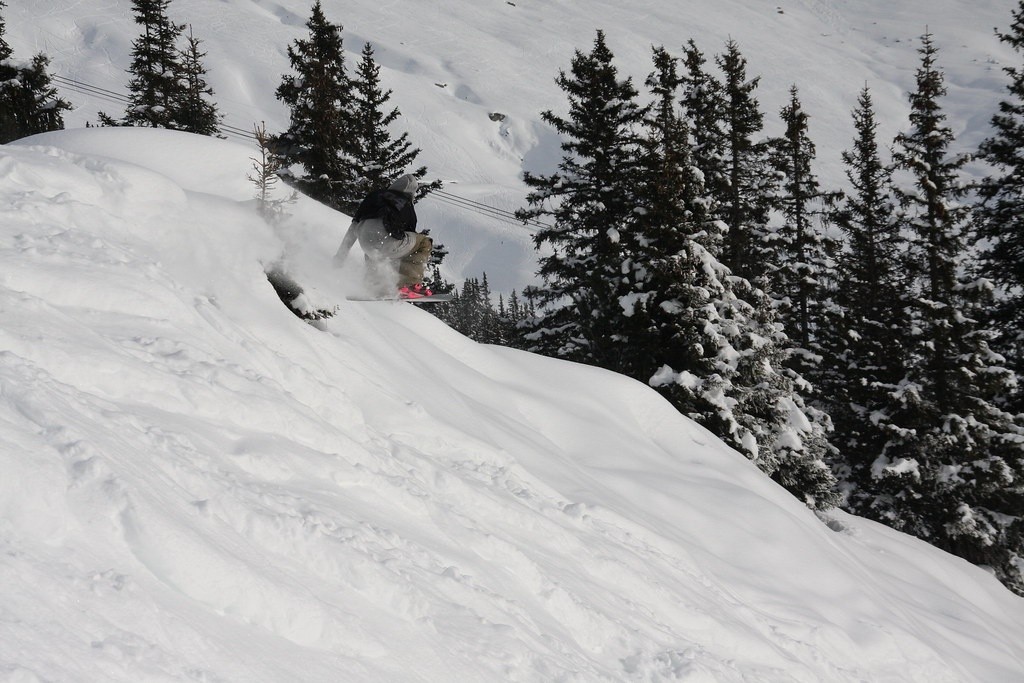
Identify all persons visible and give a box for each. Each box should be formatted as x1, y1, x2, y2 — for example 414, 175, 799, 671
329, 174, 431, 298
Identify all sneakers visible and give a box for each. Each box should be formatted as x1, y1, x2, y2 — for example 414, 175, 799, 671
398, 284, 412, 299
409, 284, 432, 299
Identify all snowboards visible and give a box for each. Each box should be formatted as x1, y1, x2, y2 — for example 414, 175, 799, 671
345, 293, 454, 302
